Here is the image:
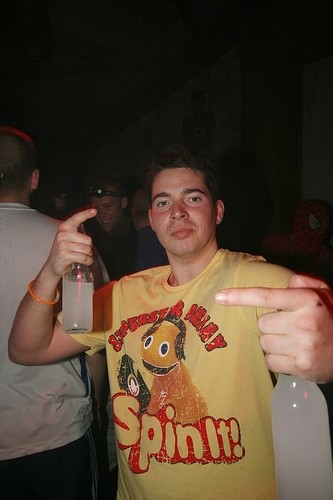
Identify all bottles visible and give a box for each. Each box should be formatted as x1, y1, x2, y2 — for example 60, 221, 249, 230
62, 220, 94, 332
271, 371, 333, 500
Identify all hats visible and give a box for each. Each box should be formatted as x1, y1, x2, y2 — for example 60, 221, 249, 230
0, 125, 37, 154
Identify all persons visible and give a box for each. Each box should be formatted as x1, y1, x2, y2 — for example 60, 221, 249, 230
258, 200, 333, 285
86, 175, 170, 281
8, 147, 333, 500
0, 126, 111, 500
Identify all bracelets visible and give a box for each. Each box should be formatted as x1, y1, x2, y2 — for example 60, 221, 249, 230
28, 281, 60, 305
